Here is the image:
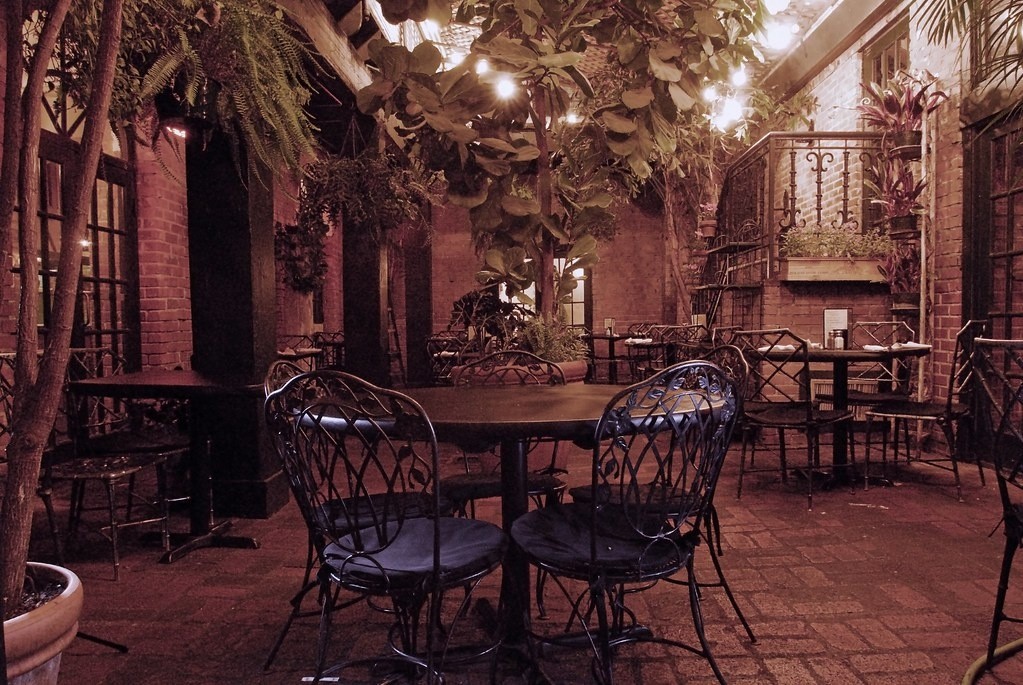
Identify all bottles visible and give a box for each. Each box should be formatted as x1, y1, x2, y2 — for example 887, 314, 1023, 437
827, 331, 835, 350
834, 330, 844, 350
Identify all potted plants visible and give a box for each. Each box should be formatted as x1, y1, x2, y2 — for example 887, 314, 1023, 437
855, 74, 951, 315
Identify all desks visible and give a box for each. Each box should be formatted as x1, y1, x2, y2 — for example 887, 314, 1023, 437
67, 369, 270, 564
578, 332, 650, 385
748, 346, 930, 487
433, 352, 481, 366
293, 384, 727, 685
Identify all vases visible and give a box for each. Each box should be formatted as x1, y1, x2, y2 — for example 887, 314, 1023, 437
698, 219, 716, 237
692, 250, 707, 265
684, 278, 701, 294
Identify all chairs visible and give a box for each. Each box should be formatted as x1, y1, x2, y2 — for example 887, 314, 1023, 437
0, 318, 1023, 685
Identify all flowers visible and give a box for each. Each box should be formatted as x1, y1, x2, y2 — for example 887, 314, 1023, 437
681, 201, 718, 295
